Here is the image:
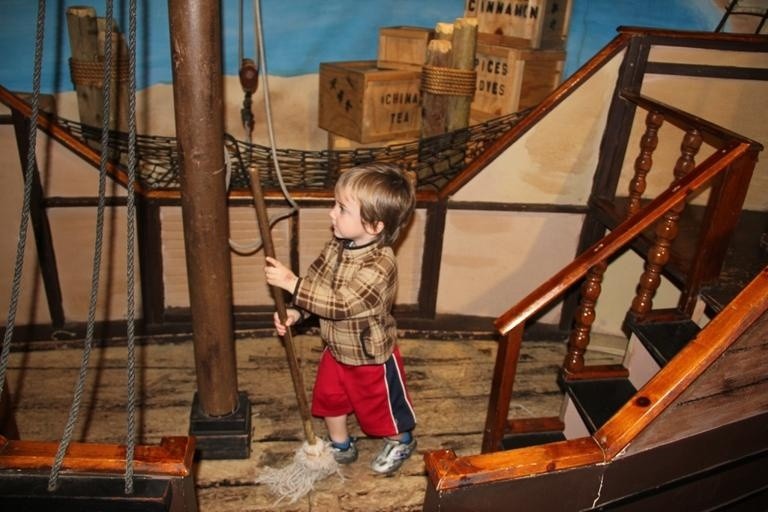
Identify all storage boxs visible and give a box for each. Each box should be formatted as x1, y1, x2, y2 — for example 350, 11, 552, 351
320, 1, 572, 192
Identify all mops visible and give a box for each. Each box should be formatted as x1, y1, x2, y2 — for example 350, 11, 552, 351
247, 163, 349, 502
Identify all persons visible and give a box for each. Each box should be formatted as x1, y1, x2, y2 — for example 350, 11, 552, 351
263, 160, 419, 477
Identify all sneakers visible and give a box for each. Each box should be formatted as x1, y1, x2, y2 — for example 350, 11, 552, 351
326, 442, 357, 464
371, 438, 417, 474
